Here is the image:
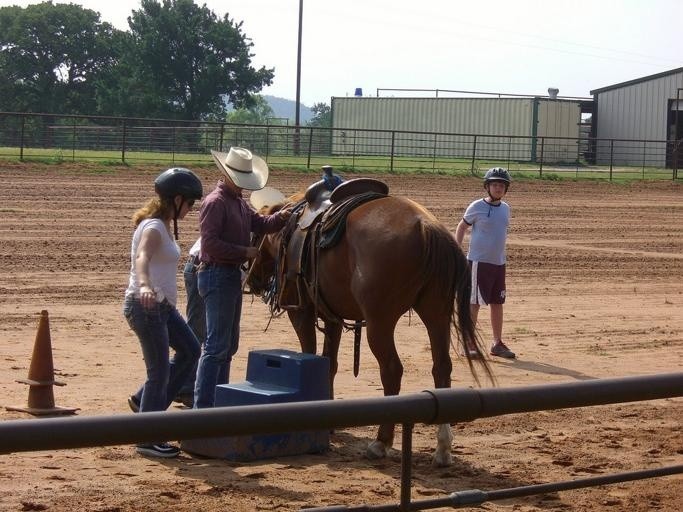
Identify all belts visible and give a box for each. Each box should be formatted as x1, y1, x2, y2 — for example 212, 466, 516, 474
188, 254, 198, 263
196, 261, 242, 270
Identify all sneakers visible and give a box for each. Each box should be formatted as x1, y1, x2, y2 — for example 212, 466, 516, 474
134, 439, 180, 458
172, 392, 193, 407
459, 347, 478, 359
127, 395, 140, 412
489, 341, 515, 359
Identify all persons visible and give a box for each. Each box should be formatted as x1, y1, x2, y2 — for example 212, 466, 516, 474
121, 167, 202, 459
455, 167, 516, 357
194, 147, 294, 408
172, 187, 290, 405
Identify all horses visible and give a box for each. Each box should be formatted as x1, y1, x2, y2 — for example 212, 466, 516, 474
243, 191, 497, 470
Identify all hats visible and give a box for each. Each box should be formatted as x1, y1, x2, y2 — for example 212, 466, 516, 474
210, 146, 268, 190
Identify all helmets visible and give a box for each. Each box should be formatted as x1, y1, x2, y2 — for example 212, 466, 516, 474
483, 167, 510, 189
154, 166, 203, 200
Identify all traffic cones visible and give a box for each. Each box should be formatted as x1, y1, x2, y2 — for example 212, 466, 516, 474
5, 309, 82, 415
